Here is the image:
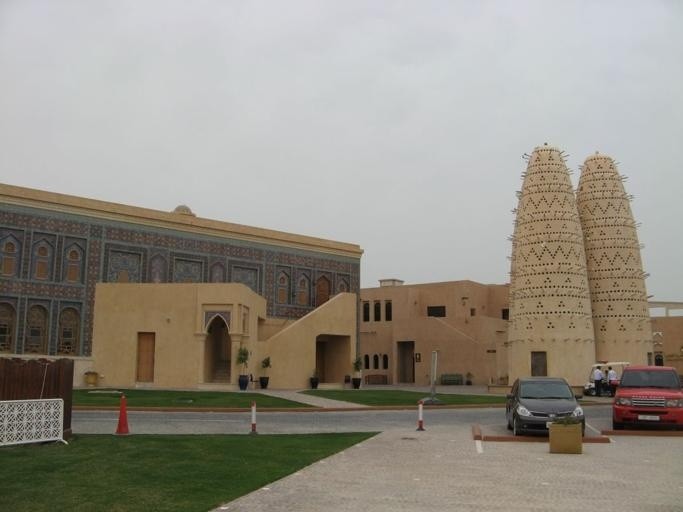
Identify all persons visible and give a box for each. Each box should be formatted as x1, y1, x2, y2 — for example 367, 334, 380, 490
591, 366, 603, 396
607, 366, 618, 397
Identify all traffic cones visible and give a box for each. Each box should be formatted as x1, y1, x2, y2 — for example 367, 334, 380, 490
112, 394, 132, 436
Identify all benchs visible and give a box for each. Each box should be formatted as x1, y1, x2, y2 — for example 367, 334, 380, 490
440, 372, 464, 385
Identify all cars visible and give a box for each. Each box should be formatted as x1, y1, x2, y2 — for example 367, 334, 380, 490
505, 376, 586, 439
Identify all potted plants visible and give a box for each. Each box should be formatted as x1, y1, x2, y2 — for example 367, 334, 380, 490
258, 355, 273, 390
351, 350, 364, 390
235, 346, 250, 392
84, 370, 100, 387
309, 367, 320, 389
548, 407, 584, 455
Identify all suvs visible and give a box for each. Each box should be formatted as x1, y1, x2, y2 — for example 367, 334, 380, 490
610, 363, 683, 429
